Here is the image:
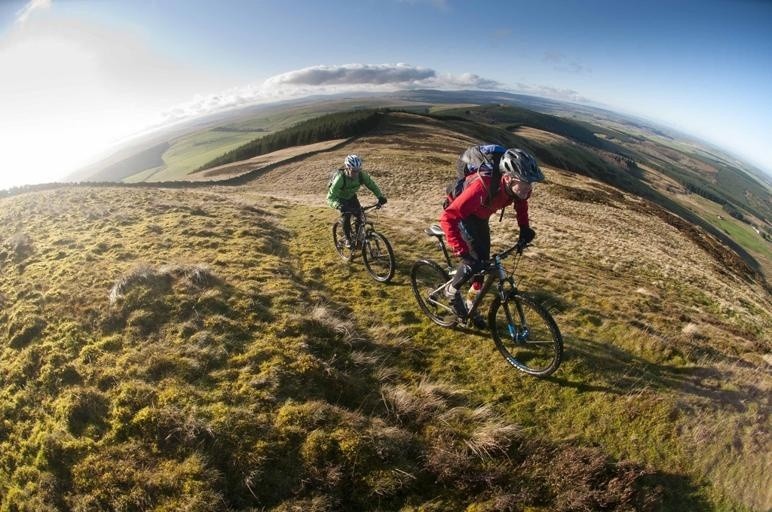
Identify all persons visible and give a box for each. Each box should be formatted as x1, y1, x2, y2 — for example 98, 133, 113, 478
440, 147, 544, 329
325, 155, 387, 249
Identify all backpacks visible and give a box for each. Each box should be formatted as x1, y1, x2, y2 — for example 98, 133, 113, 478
456, 144, 505, 208
328, 168, 363, 191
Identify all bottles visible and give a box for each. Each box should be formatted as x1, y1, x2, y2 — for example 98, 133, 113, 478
465, 282, 482, 314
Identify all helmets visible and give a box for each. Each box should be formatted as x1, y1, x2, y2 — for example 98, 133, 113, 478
345, 154, 361, 170
498, 148, 544, 183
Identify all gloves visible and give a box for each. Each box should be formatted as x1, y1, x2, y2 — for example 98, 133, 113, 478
379, 197, 387, 205
520, 226, 536, 244
337, 205, 347, 212
460, 254, 477, 268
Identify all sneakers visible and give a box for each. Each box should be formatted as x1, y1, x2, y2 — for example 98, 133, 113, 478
465, 300, 486, 331
345, 239, 351, 247
444, 286, 467, 318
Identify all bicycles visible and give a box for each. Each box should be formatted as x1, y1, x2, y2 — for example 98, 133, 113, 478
332, 202, 396, 283
410, 224, 564, 377
